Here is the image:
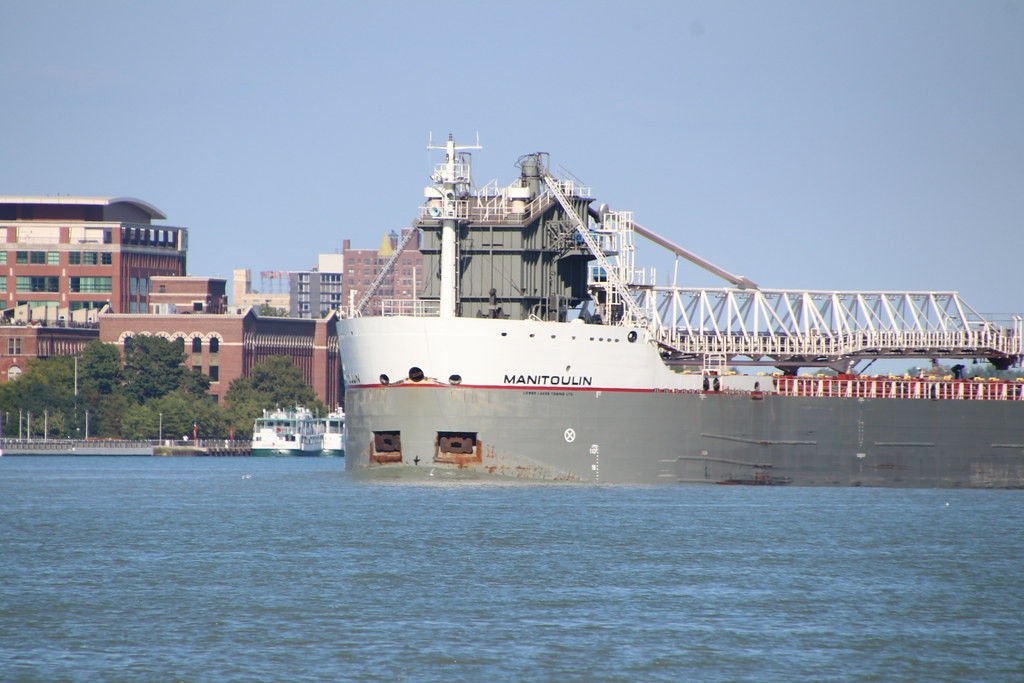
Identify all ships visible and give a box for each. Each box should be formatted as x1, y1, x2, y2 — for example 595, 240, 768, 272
334, 130, 1023, 492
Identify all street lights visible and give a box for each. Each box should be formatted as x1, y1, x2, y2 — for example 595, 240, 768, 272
158, 412, 162, 446
26, 410, 30, 439
85, 409, 89, 440
44, 408, 48, 442
19, 408, 23, 440
5, 412, 9, 424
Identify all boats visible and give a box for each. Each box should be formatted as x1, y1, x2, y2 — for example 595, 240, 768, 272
250, 403, 325, 456
322, 402, 346, 458
